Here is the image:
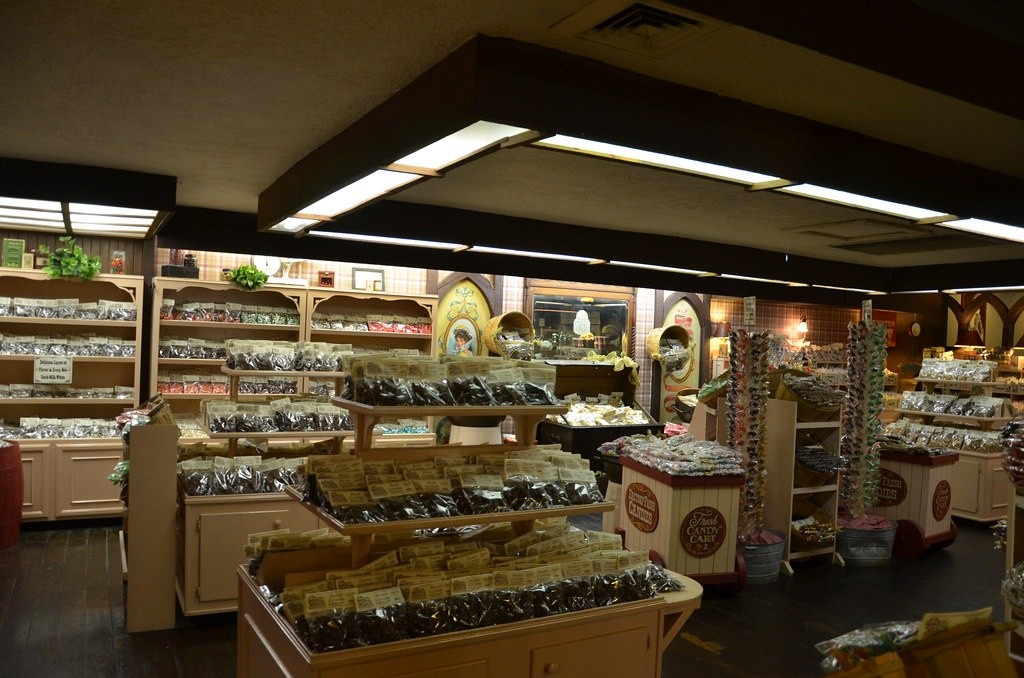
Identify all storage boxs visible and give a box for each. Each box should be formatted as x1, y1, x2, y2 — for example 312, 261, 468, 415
161, 265, 182, 278
182, 267, 199, 278
318, 272, 335, 288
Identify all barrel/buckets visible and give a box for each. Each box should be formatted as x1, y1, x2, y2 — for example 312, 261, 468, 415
737, 528, 786, 584
837, 517, 898, 567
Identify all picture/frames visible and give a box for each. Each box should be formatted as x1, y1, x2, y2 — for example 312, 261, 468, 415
22, 253, 34, 269
352, 267, 385, 291
2, 238, 25, 268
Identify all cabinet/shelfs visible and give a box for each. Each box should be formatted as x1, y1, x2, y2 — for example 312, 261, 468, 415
716, 396, 845, 575
886, 378, 1014, 528
150, 276, 308, 447
119, 424, 178, 633
0, 267, 144, 522
178, 364, 383, 626
236, 396, 703, 678
1003, 477, 1024, 663
304, 286, 439, 448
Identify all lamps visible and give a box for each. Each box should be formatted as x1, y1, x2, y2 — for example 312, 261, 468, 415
791, 315, 809, 332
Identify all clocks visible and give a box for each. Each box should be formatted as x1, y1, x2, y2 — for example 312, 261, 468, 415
250, 255, 281, 277
909, 322, 922, 338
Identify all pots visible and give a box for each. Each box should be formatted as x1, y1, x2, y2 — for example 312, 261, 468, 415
671, 388, 700, 423
